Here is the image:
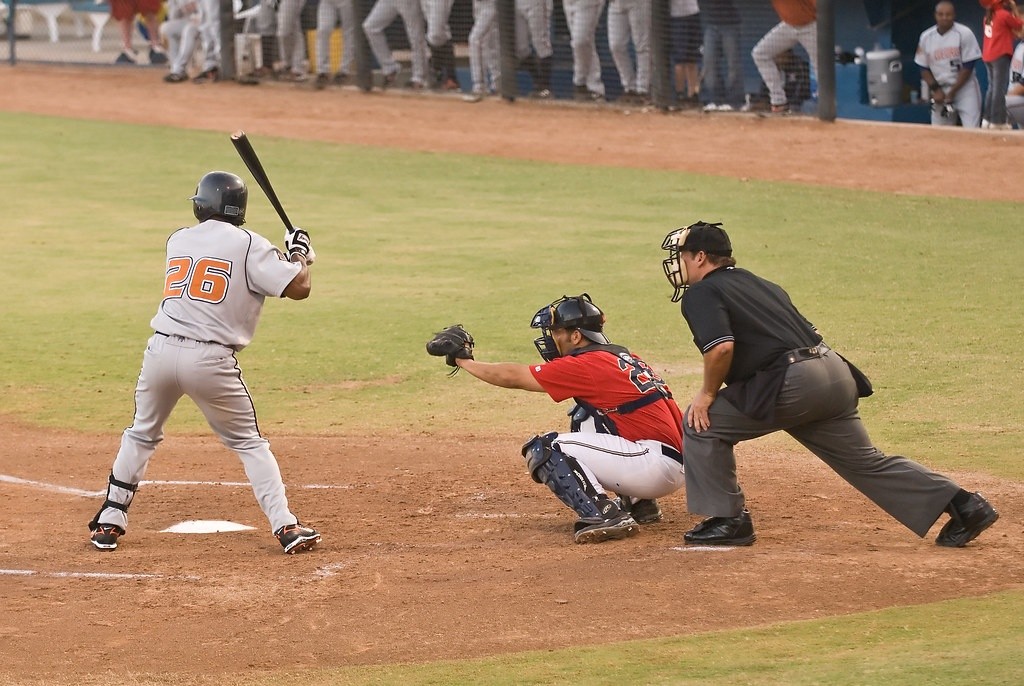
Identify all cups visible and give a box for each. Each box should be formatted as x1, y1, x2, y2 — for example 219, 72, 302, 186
910, 92, 918, 104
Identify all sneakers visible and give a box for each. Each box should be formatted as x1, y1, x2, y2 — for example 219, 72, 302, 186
574, 509, 640, 544
685, 509, 755, 546
90, 524, 122, 551
935, 491, 999, 548
613, 494, 664, 525
275, 524, 322, 555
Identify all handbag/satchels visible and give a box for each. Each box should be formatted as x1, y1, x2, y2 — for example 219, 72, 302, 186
718, 362, 790, 421
835, 351, 874, 398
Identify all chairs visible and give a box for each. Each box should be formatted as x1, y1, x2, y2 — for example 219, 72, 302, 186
0, 0, 112, 53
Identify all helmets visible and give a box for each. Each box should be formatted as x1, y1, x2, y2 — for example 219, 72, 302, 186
554, 298, 612, 346
192, 170, 248, 225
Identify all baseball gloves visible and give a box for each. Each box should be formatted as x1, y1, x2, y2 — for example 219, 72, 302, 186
426, 325, 475, 367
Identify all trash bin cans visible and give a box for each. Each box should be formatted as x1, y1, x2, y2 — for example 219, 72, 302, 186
866, 47, 905, 107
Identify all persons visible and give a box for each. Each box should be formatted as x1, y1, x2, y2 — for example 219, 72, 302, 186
88, 171, 323, 555
105, 0, 224, 85
660, 221, 1001, 548
237, 1, 817, 115
907, 0, 1023, 130
425, 293, 685, 543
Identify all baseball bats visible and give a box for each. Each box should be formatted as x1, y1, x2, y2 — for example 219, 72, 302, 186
229, 129, 313, 266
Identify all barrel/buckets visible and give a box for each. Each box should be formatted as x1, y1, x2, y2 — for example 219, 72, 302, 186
866, 49, 903, 107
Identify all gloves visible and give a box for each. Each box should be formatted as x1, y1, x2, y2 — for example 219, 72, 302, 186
284, 227, 311, 258
283, 246, 315, 266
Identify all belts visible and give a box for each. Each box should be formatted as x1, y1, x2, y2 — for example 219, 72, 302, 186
661, 445, 683, 466
787, 343, 830, 364
155, 331, 170, 337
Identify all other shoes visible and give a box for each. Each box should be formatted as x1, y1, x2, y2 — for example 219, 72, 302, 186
116, 44, 819, 120
981, 119, 1012, 130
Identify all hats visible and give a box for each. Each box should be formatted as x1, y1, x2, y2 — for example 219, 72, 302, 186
672, 221, 732, 256
